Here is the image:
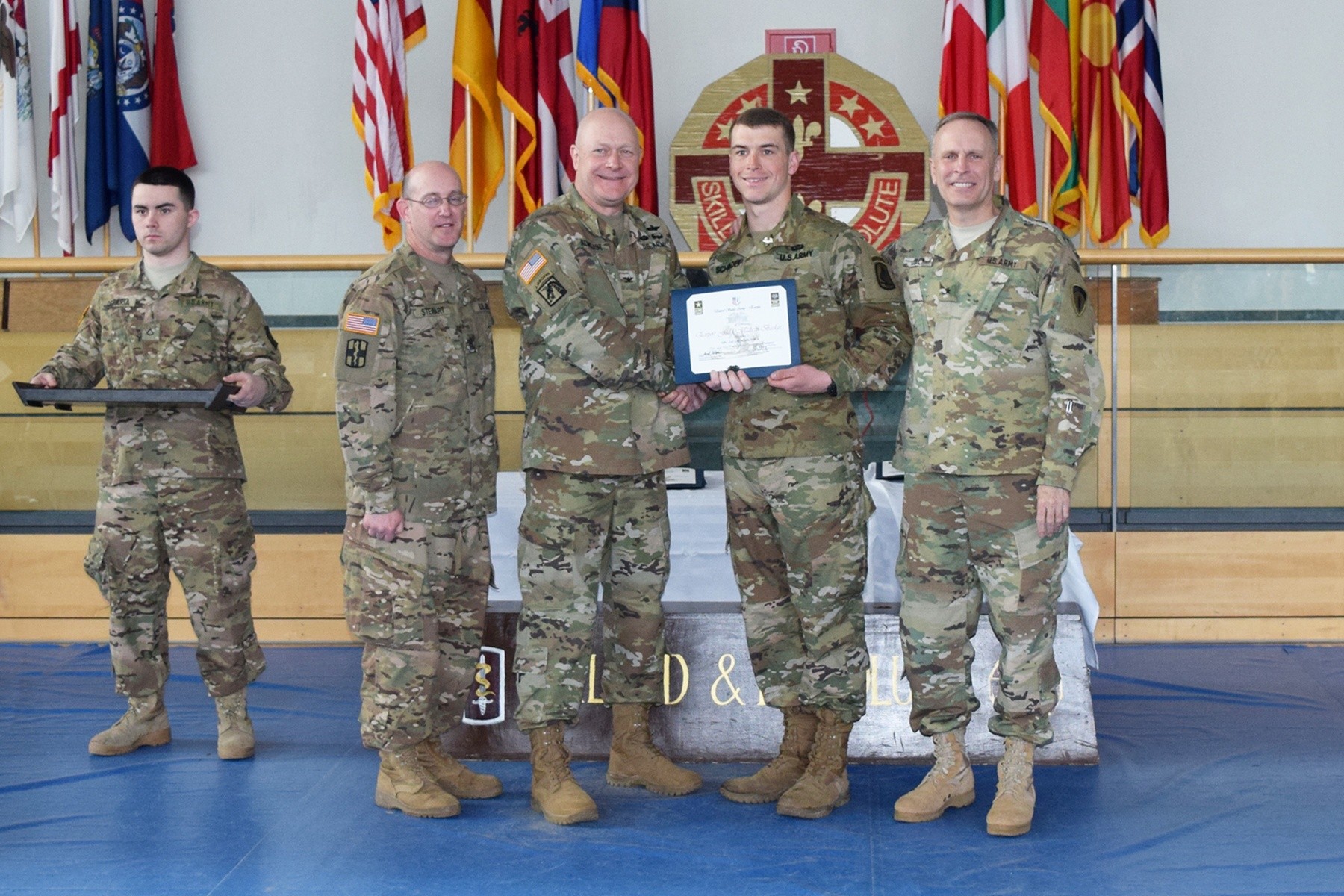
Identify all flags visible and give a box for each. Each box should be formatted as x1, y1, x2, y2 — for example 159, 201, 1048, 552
937, 0, 1170, 251
353, 0, 659, 255
0, 0, 199, 263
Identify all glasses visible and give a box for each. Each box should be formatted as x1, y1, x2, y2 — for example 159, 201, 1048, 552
402, 192, 468, 209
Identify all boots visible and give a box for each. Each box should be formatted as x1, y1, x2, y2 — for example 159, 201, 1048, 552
606, 702, 703, 795
216, 685, 255, 758
88, 688, 172, 755
892, 726, 975, 823
985, 737, 1035, 835
719, 709, 817, 803
529, 728, 598, 825
777, 708, 853, 818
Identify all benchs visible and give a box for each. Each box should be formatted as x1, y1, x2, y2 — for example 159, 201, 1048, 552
439, 600, 1101, 766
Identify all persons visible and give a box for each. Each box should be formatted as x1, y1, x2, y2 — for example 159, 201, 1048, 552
501, 108, 704, 824
28, 166, 293, 761
334, 160, 501, 819
706, 107, 913, 820
731, 112, 1104, 837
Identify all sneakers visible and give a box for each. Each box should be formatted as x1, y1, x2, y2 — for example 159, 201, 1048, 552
376, 748, 461, 818
417, 738, 502, 798
